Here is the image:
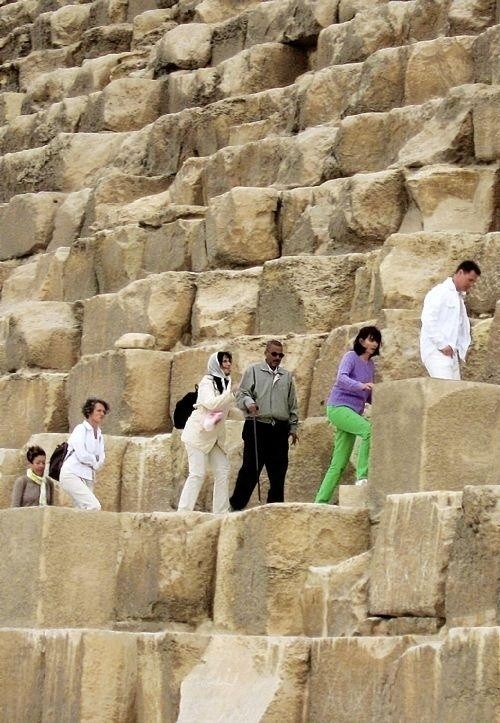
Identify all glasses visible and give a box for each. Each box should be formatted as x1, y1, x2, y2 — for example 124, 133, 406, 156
223, 359, 232, 363
267, 350, 285, 358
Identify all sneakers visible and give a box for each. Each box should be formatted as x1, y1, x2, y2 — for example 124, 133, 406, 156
356, 479, 367, 485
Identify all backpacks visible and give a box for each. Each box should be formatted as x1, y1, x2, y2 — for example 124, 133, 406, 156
48, 442, 68, 482
174, 384, 204, 430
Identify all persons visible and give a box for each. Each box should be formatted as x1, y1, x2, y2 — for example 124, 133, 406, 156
313, 324, 383, 504
175, 349, 247, 514
59, 396, 111, 511
9, 442, 54, 508
228, 337, 300, 513
420, 258, 482, 381
